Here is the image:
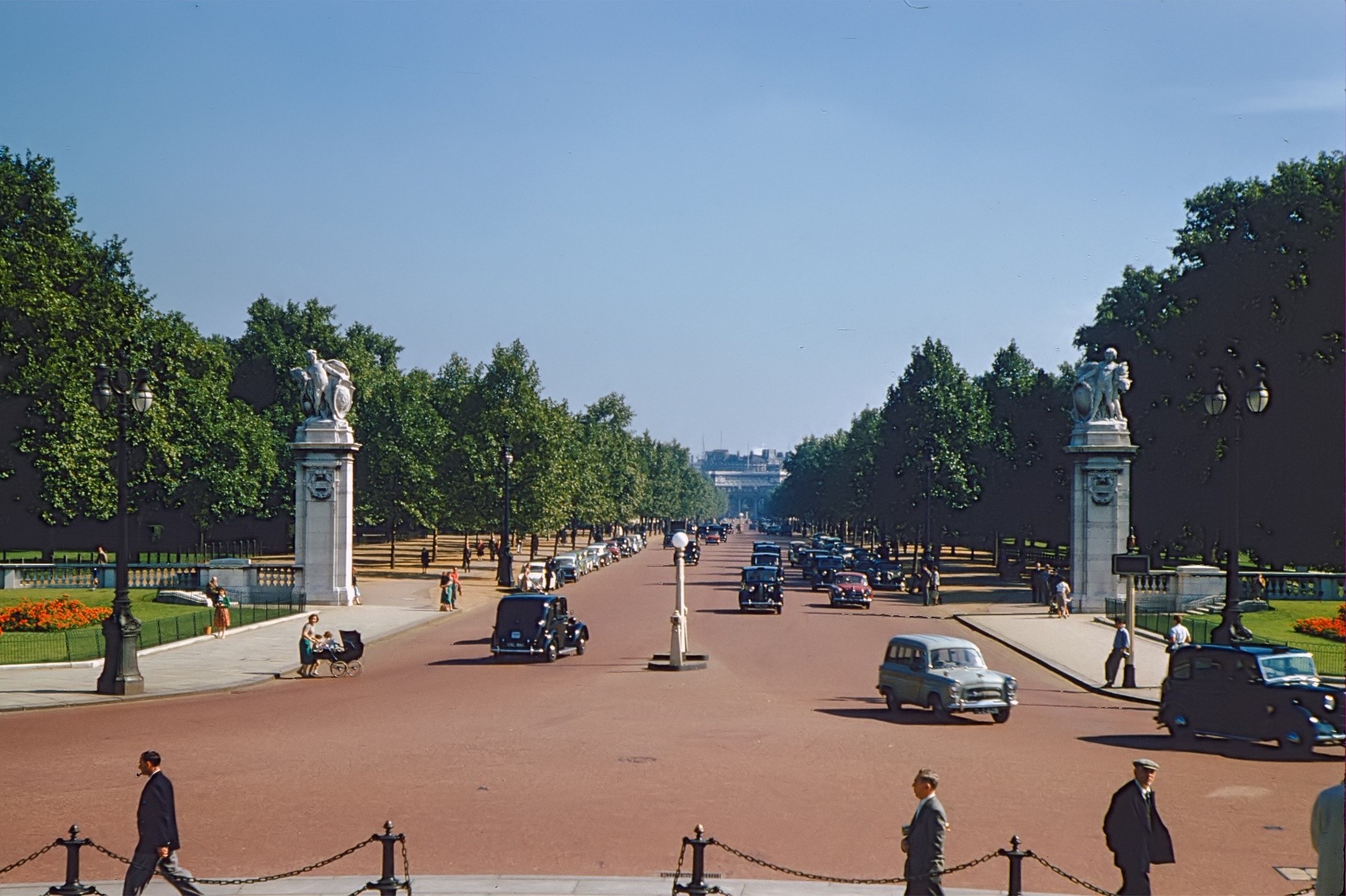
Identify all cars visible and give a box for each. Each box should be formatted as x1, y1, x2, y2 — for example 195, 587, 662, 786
751, 540, 783, 573
799, 550, 831, 580
788, 532, 907, 592
551, 557, 580, 583
748, 515, 783, 537
875, 633, 1019, 723
738, 566, 783, 614
490, 594, 590, 663
1152, 643, 1346, 758
558, 530, 648, 577
673, 540, 700, 566
518, 561, 557, 591
828, 572, 873, 608
661, 519, 739, 549
811, 555, 847, 590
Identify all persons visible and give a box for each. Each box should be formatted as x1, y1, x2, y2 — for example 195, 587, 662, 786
122, 750, 205, 896
515, 535, 523, 553
1170, 615, 1192, 652
520, 563, 532, 592
1056, 577, 1071, 619
1311, 772, 1343, 896
931, 566, 943, 605
476, 539, 485, 561
421, 547, 429, 574
533, 534, 539, 555
296, 614, 336, 677
463, 543, 473, 573
206, 577, 231, 639
440, 571, 454, 612
1103, 760, 1176, 896
92, 547, 107, 585
559, 530, 569, 546
451, 567, 462, 610
1030, 562, 1062, 606
352, 566, 363, 604
921, 564, 932, 606
488, 534, 501, 562
544, 556, 554, 594
900, 769, 947, 896
1099, 617, 1130, 689
535, 582, 547, 593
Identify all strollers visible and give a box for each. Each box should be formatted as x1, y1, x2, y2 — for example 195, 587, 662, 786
1048, 594, 1058, 615
529, 578, 550, 595
314, 629, 365, 677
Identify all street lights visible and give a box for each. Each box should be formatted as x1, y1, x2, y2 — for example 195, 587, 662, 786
923, 446, 935, 563
667, 532, 689, 667
498, 431, 515, 587
89, 361, 157, 694
1206, 360, 1271, 642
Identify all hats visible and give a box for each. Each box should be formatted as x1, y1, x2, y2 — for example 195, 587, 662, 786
1132, 759, 1160, 770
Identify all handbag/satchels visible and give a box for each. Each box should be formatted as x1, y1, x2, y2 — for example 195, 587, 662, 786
930, 590, 940, 599
439, 604, 448, 611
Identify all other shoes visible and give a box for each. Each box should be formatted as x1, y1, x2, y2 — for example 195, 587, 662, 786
297, 671, 307, 677
1100, 681, 1114, 688
309, 672, 318, 677
